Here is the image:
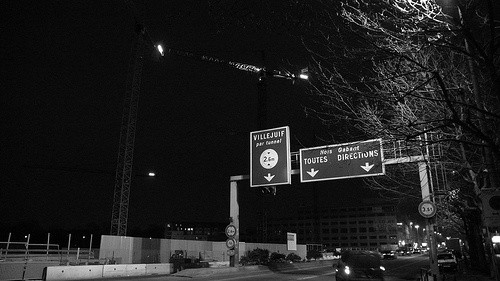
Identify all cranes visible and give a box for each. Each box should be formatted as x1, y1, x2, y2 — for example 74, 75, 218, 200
108, 20, 309, 236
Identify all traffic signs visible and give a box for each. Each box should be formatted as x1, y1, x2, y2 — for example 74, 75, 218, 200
250, 126, 292, 187
300, 139, 387, 181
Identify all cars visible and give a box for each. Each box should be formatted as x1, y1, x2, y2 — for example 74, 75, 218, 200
335, 249, 384, 281
383, 244, 430, 259
436, 253, 456, 270
